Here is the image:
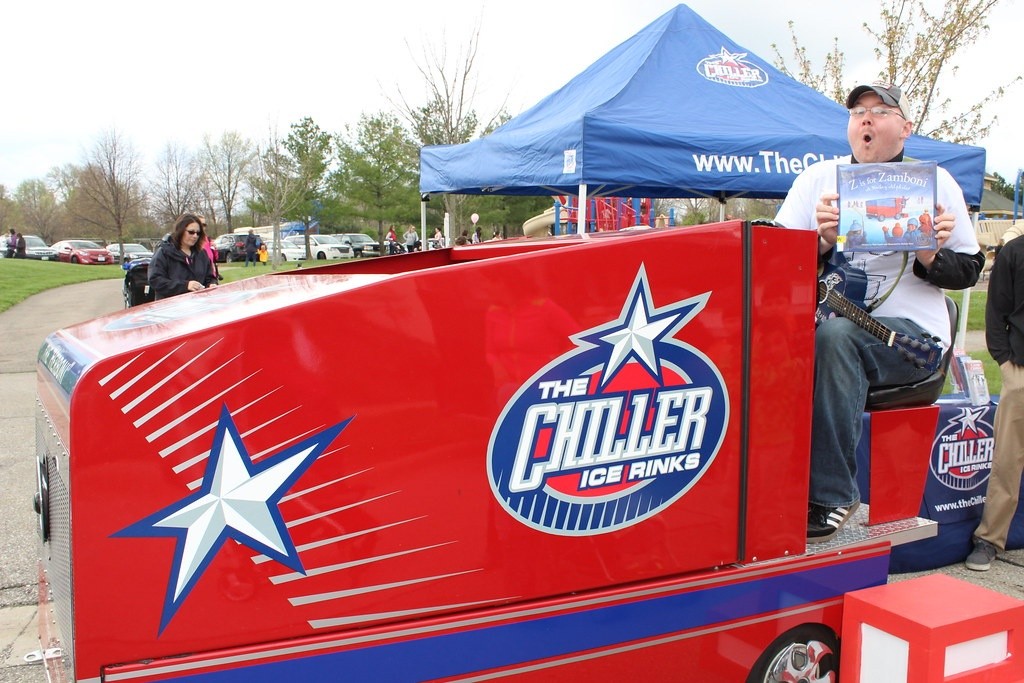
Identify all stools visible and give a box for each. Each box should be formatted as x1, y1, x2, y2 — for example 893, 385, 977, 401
840, 572, 1024, 683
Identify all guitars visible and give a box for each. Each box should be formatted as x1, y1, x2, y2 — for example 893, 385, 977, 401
751, 217, 944, 372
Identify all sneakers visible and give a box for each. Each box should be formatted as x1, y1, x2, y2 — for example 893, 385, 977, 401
964, 537, 995, 571
806, 498, 861, 542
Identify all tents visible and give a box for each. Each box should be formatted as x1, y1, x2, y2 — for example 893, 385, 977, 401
420, 4, 986, 250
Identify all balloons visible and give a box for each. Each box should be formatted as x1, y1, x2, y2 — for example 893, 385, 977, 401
471, 213, 479, 225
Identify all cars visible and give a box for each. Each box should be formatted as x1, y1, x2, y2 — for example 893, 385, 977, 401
213, 234, 267, 263
0, 235, 59, 261
105, 243, 154, 263
331, 233, 380, 258
284, 235, 355, 260
50, 240, 114, 265
263, 241, 307, 260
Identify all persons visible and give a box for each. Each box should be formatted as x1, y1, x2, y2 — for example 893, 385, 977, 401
979, 238, 1005, 283
546, 224, 576, 237
965, 234, 1024, 571
3, 229, 26, 258
197, 214, 223, 280
772, 81, 986, 543
594, 198, 632, 234
403, 225, 419, 253
432, 227, 443, 249
385, 224, 397, 255
149, 213, 213, 300
241, 229, 268, 267
455, 226, 503, 246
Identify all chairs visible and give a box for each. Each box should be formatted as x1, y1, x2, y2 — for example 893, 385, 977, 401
865, 293, 960, 413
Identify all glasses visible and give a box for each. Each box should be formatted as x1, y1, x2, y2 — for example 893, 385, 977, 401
847, 107, 906, 121
203, 223, 207, 227
185, 230, 200, 236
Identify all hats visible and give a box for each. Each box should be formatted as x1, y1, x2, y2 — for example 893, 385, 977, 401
846, 81, 911, 121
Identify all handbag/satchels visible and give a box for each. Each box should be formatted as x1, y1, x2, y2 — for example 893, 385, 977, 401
414, 241, 421, 246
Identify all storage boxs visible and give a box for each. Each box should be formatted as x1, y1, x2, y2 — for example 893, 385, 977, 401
836, 160, 938, 252
